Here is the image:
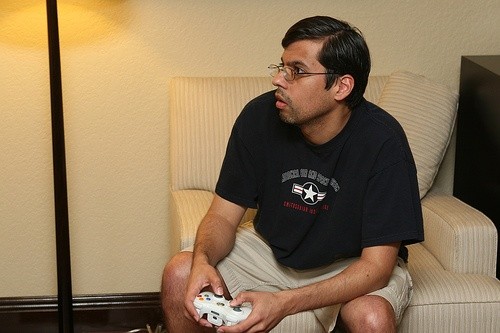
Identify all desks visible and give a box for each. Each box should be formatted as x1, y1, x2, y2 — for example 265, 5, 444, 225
452, 55, 500, 281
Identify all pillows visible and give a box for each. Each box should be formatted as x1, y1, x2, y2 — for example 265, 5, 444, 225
377, 70, 459, 200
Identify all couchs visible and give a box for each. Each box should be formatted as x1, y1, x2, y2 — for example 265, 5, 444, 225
169, 70, 500, 333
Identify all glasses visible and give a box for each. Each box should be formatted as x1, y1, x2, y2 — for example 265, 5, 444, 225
266, 64, 343, 80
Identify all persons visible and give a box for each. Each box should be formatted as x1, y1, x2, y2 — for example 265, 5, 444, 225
160, 14, 425, 333
